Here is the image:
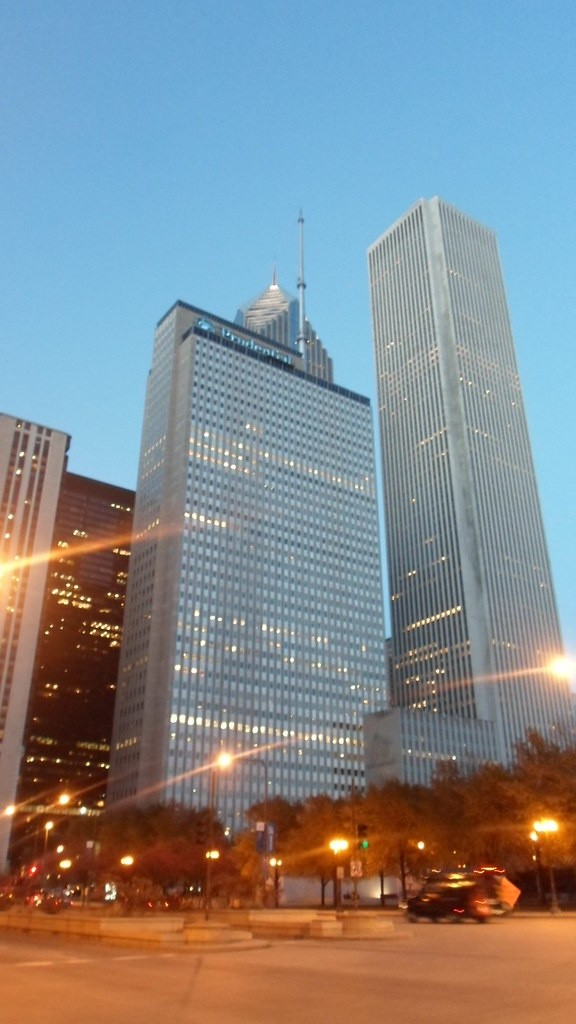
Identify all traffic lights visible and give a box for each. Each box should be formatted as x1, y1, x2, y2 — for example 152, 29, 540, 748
354, 824, 371, 850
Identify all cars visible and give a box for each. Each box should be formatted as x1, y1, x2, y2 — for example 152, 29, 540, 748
0, 883, 74, 912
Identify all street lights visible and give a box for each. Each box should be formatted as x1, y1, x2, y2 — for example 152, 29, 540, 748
203, 753, 233, 918
536, 814, 562, 913
331, 836, 350, 911
